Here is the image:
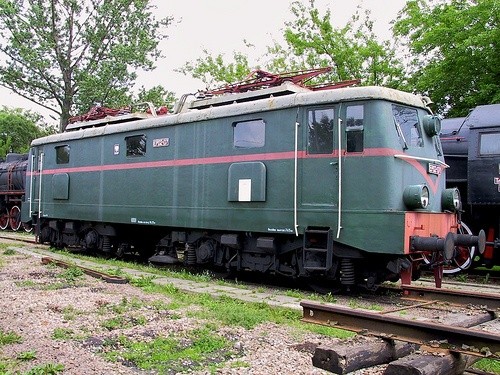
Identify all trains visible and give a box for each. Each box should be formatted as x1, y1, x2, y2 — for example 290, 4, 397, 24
20, 66, 486, 295
421, 103, 500, 277
0, 151, 36, 232
